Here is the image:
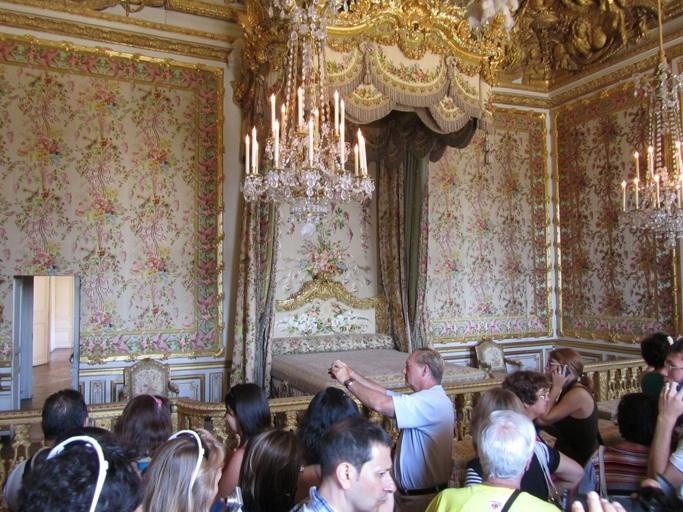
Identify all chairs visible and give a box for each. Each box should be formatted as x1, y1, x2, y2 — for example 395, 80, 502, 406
122, 358, 180, 401
473, 338, 524, 379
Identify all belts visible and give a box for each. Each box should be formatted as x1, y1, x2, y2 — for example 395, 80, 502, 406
398, 482, 449, 497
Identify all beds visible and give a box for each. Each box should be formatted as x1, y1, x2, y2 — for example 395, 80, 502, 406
262, 269, 489, 430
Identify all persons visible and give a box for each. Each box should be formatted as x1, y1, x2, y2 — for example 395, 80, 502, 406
135, 428, 225, 512
241, 428, 303, 512
18, 427, 145, 512
462, 387, 584, 503
576, 393, 672, 496
425, 410, 562, 512
641, 332, 674, 372
647, 338, 683, 502
502, 371, 566, 452
570, 491, 626, 512
294, 387, 359, 503
112, 395, 172, 475
0, 389, 90, 511
291, 416, 396, 512
328, 348, 454, 511
545, 348, 604, 491
214, 384, 272, 498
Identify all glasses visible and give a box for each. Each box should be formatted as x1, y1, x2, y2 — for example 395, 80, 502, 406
538, 393, 547, 400
663, 361, 683, 372
168, 429, 209, 492
46, 433, 114, 512
547, 361, 569, 368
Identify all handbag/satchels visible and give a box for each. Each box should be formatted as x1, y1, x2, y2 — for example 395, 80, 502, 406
535, 442, 566, 511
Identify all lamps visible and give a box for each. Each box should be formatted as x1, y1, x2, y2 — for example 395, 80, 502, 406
239, 0, 375, 237
618, 48, 683, 249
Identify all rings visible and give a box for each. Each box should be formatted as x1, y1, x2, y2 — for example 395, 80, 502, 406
558, 371, 562, 375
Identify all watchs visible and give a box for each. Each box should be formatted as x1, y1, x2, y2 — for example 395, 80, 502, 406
344, 378, 356, 388
